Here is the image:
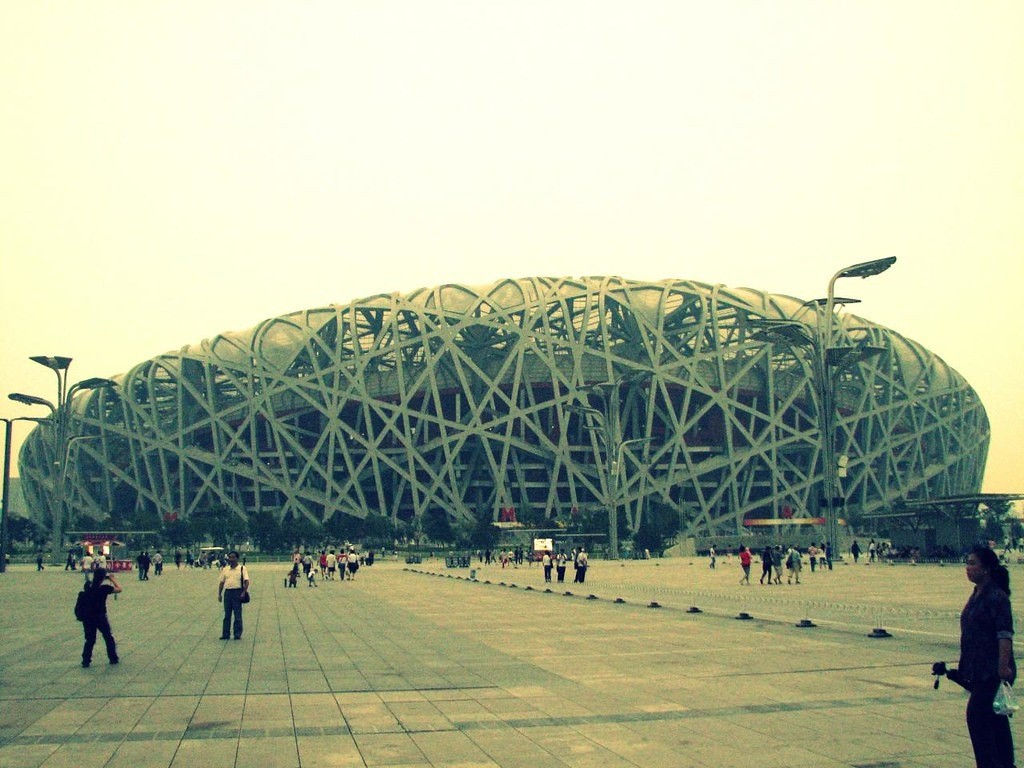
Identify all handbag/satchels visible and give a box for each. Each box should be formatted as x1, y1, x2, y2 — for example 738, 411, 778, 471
992, 679, 1019, 716
786, 551, 793, 569
241, 591, 250, 603
338, 563, 341, 568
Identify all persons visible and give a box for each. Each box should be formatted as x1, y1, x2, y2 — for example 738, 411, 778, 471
287, 543, 651, 588
5, 549, 250, 667
958, 546, 1018, 768
712, 536, 1024, 585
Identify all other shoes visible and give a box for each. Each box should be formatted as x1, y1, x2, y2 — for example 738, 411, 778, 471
110, 660, 119, 664
788, 580, 791, 585
234, 636, 240, 639
747, 582, 751, 585
220, 636, 230, 639
760, 578, 763, 584
774, 578, 777, 585
739, 580, 743, 586
779, 580, 782, 584
796, 581, 800, 583
768, 581, 772, 584
83, 663, 90, 667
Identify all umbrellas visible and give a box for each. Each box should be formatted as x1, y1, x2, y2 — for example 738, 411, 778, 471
77, 540, 125, 560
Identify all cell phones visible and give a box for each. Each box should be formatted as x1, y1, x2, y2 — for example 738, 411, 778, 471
105, 576, 109, 578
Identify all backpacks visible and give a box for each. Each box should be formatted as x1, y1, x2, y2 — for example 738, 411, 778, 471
75, 590, 91, 621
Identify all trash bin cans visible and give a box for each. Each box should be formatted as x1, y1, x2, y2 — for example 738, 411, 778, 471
445, 557, 459, 567
459, 556, 470, 567
406, 557, 414, 563
414, 557, 422, 563
